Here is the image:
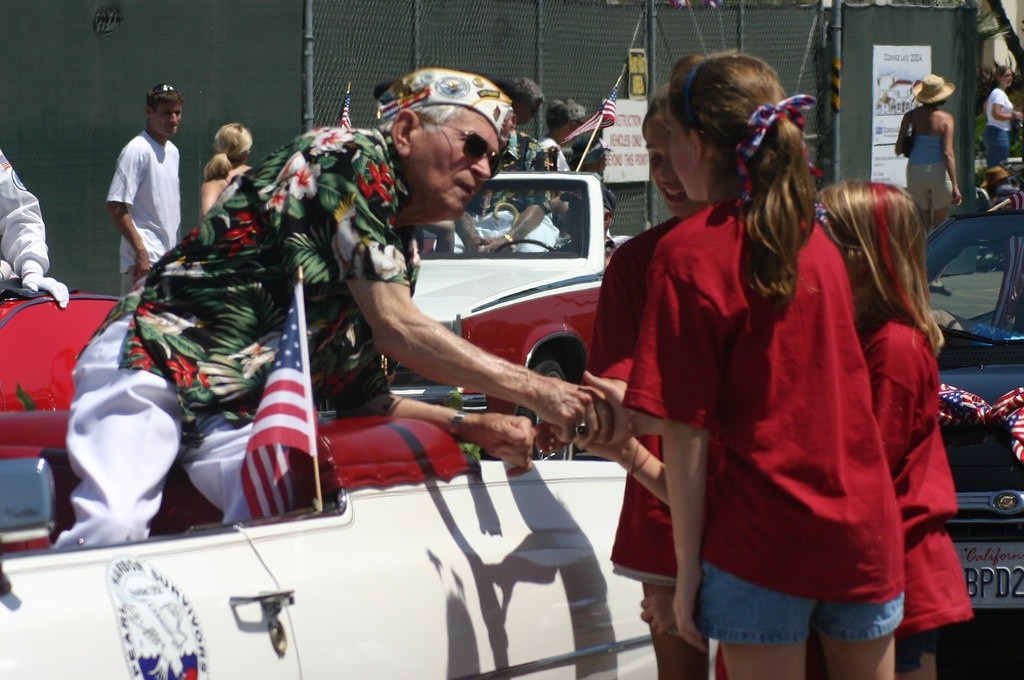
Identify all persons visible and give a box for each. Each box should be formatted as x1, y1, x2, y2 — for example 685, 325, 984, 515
54, 68, 613, 552
198, 123, 254, 221
106, 83, 183, 295
0, 150, 70, 308
417, 50, 1024, 680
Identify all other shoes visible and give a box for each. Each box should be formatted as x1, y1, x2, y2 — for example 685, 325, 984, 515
929, 285, 952, 297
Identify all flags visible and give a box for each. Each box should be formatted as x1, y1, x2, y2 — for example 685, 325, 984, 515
562, 86, 619, 145
239, 282, 338, 519
1009, 190, 1024, 210
338, 88, 353, 129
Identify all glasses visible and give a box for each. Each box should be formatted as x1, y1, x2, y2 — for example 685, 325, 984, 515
444, 124, 502, 181
152, 84, 177, 96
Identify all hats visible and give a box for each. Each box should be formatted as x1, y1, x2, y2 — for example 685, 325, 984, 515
569, 136, 611, 166
560, 180, 617, 212
912, 74, 955, 103
375, 67, 517, 153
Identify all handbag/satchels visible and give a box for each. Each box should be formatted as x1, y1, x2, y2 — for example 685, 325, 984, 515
902, 110, 915, 158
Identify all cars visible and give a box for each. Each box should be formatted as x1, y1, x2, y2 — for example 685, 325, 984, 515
0, 166, 1023, 680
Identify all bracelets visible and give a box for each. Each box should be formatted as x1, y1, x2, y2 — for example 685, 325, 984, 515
628, 453, 652, 476
450, 411, 466, 445
503, 235, 516, 248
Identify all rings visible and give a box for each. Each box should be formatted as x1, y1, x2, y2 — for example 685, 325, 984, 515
575, 421, 589, 435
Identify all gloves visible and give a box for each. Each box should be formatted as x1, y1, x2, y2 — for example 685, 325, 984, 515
22, 273, 69, 308
